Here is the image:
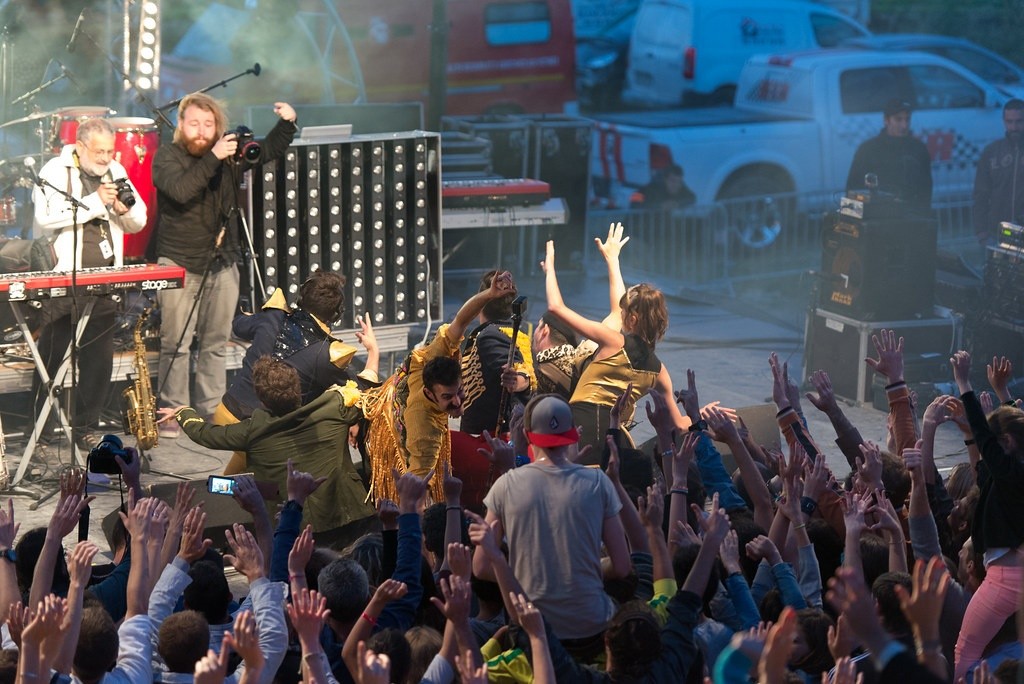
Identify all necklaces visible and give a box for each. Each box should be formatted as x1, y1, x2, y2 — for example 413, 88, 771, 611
534, 457, 568, 462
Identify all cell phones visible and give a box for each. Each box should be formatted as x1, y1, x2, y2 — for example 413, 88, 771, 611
206, 475, 239, 496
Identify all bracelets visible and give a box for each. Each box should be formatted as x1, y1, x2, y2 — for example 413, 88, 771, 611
915, 639, 942, 655
445, 505, 461, 511
661, 450, 674, 456
361, 612, 377, 624
19, 672, 39, 679
799, 496, 818, 517
964, 439, 976, 445
670, 486, 689, 490
793, 523, 805, 530
289, 575, 306, 580
670, 489, 688, 495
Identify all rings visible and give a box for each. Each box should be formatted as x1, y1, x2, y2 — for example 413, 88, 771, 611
185, 527, 190, 530
153, 513, 158, 517
528, 604, 534, 609
61, 508, 66, 512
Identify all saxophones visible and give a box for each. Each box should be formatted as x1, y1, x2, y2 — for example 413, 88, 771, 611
121, 307, 160, 450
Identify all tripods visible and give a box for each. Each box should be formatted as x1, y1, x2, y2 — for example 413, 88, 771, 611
29, 176, 128, 510
154, 163, 268, 418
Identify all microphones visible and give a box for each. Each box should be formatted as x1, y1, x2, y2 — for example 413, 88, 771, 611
67, 12, 84, 52
23, 157, 45, 194
55, 59, 80, 86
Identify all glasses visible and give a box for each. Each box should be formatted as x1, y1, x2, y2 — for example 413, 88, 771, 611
83, 144, 116, 159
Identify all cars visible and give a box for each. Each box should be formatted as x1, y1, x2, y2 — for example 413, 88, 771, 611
573, 7, 639, 91
570, 0, 643, 39
835, 33, 1024, 102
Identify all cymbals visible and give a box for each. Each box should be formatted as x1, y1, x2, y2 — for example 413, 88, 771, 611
1, 111, 55, 128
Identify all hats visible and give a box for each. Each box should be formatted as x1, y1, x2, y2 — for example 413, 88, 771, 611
543, 311, 578, 347
523, 393, 580, 447
884, 95, 914, 116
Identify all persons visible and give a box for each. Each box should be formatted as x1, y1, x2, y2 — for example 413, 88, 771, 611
150, 93, 297, 436
846, 97, 934, 208
0, 223, 1024, 684
22, 119, 147, 452
971, 99, 1024, 245
636, 164, 696, 212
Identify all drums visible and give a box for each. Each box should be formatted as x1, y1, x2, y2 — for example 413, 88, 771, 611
0, 196, 17, 226
48, 106, 162, 261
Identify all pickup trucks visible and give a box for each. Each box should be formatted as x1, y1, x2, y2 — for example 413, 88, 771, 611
577, 48, 1009, 255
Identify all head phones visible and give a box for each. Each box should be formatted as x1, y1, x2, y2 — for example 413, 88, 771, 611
296, 277, 346, 322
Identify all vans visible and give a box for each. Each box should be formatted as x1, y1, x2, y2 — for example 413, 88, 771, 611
619, 0, 874, 112
159, 1, 575, 116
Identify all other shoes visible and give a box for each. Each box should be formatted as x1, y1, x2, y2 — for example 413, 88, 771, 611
156, 416, 180, 438
206, 414, 215, 424
20, 443, 58, 464
77, 434, 99, 450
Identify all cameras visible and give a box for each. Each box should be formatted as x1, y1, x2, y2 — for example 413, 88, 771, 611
89, 434, 133, 474
224, 125, 263, 164
113, 178, 136, 208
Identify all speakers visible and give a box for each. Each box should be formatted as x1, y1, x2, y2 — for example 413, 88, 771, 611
101, 472, 257, 567
635, 404, 782, 486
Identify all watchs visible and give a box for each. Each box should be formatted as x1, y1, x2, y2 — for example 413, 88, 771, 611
0, 548, 16, 562
689, 420, 708, 432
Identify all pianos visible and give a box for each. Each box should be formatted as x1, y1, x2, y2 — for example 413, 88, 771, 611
442, 177, 570, 294
0, 263, 187, 488
